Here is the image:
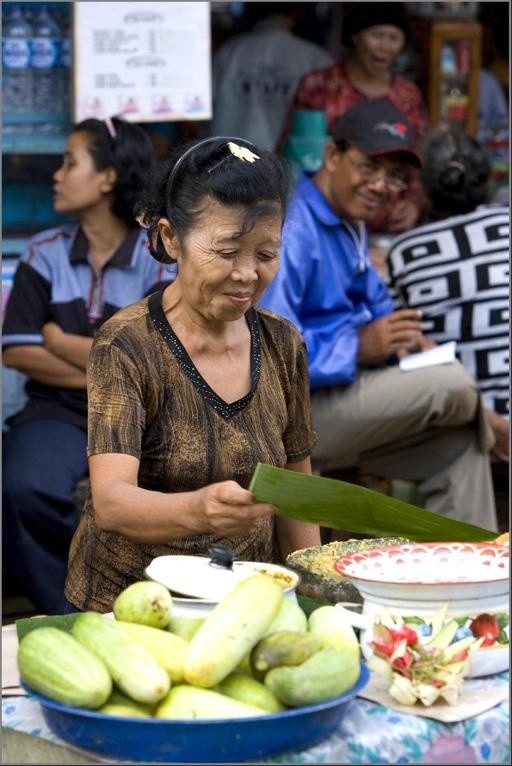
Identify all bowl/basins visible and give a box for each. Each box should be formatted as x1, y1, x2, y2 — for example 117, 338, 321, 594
333, 540, 510, 679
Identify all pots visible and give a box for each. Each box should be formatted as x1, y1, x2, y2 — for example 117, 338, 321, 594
145, 545, 310, 636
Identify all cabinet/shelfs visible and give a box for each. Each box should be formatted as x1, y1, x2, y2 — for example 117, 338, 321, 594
428, 21, 481, 144
1, 622, 512, 766
1, 112, 69, 429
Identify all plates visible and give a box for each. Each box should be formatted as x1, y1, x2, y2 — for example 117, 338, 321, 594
20, 662, 371, 764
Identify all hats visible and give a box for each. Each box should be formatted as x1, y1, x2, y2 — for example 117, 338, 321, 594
331, 96, 424, 169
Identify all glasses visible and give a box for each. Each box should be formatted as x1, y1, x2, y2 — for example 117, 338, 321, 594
343, 152, 408, 192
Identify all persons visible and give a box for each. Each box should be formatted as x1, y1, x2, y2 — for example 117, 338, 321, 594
195, 2, 336, 153
253, 99, 510, 542
1, 117, 177, 611
476, 63, 510, 162
383, 131, 509, 491
276, 1, 434, 233
59, 138, 322, 611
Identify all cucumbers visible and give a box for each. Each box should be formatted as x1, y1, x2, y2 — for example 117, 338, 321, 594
186, 570, 283, 687
222, 674, 283, 713
249, 628, 317, 682
18, 627, 112, 710
120, 624, 186, 685
265, 605, 360, 702
115, 580, 172, 625
156, 686, 265, 720
72, 613, 169, 704
166, 616, 203, 641
102, 698, 149, 720
269, 600, 307, 635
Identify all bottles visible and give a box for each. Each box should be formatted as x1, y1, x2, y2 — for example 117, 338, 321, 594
2, 1, 35, 30
26, 5, 62, 135
61, 6, 73, 135
1, 5, 33, 135
